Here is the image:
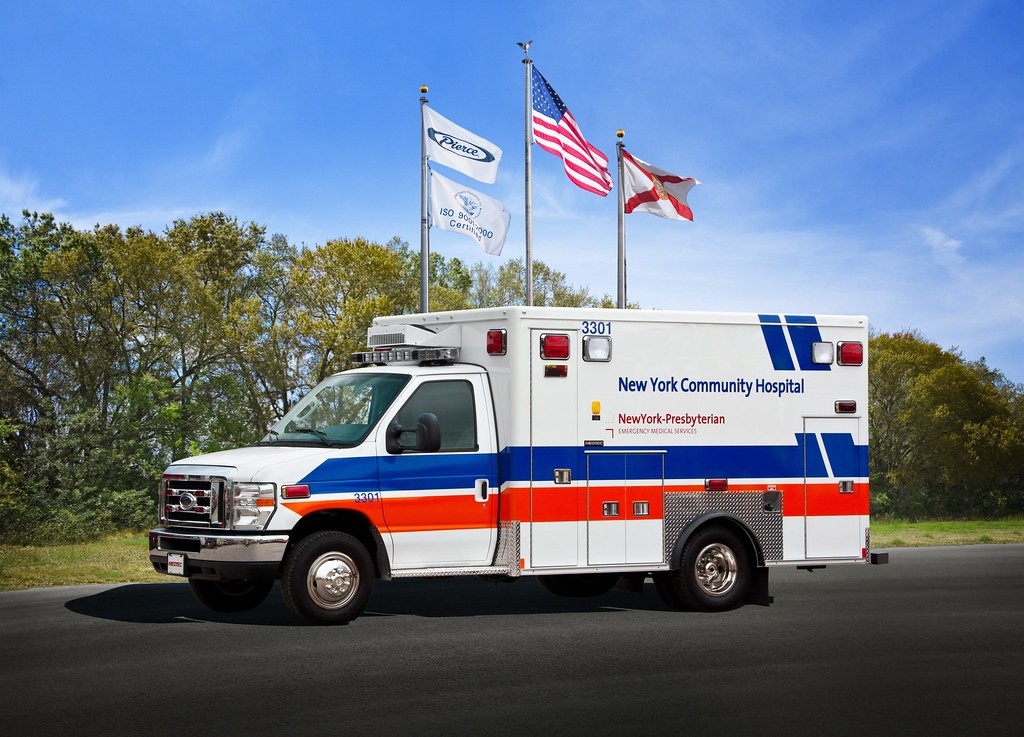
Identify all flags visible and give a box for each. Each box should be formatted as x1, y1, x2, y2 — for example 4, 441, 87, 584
429, 168, 512, 255
530, 61, 615, 198
621, 147, 705, 222
422, 102, 502, 185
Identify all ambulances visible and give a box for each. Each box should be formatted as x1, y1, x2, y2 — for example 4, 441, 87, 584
146, 306, 892, 623
